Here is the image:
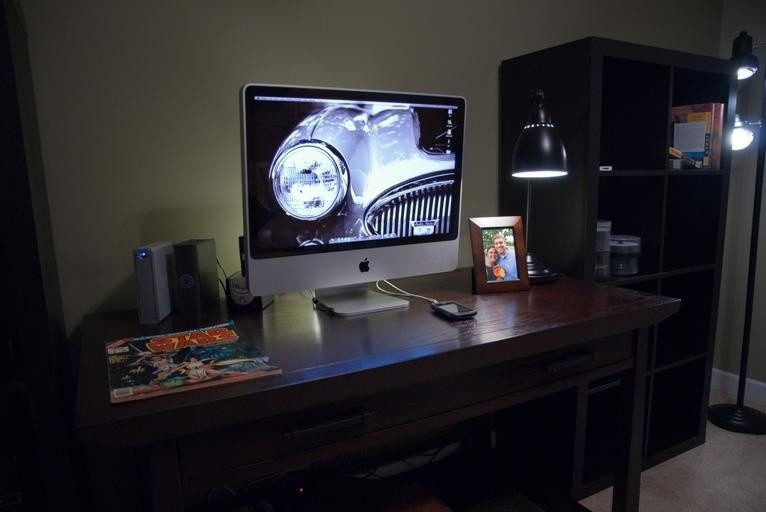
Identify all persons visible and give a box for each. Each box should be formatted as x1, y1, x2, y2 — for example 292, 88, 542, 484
485, 245, 502, 281
492, 235, 518, 280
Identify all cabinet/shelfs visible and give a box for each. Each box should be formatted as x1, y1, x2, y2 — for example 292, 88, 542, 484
501, 34, 739, 497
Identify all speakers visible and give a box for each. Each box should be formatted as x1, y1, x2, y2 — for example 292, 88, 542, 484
172, 237, 221, 315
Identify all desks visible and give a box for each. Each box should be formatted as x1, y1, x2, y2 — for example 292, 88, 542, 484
72, 269, 682, 512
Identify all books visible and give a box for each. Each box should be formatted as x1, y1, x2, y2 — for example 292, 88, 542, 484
668, 101, 725, 170
103, 319, 283, 404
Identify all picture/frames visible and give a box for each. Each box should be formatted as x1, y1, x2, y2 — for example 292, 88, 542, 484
468, 215, 528, 295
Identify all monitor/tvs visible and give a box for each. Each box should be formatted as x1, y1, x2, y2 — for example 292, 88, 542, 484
240, 82, 468, 318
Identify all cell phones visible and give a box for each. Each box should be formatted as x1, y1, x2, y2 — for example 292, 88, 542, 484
431, 300, 478, 321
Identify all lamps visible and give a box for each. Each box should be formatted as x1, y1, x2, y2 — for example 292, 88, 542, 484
724, 114, 765, 152
510, 84, 570, 285
728, 30, 766, 80
